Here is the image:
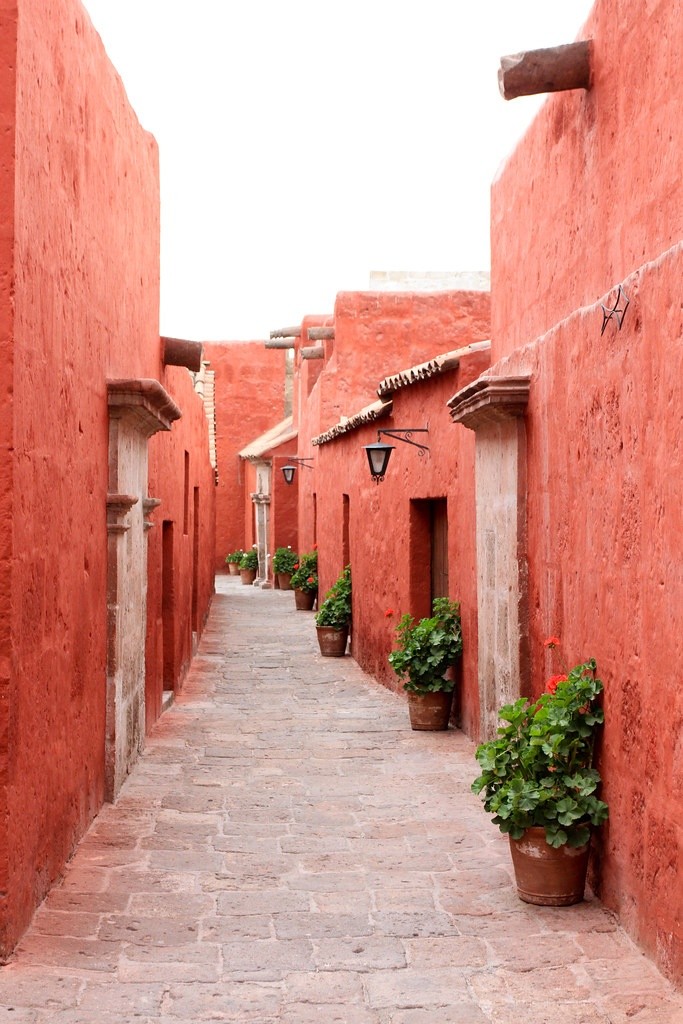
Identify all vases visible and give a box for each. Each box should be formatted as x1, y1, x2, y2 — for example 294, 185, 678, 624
294, 587, 316, 611
316, 625, 349, 657
228, 562, 240, 576
239, 569, 256, 585
277, 573, 293, 590
508, 819, 593, 906
408, 687, 452, 730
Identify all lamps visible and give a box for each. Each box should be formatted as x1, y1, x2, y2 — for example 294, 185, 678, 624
280, 457, 314, 484
361, 420, 429, 486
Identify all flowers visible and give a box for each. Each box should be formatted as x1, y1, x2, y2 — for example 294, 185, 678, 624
469, 635, 609, 848
313, 564, 352, 631
289, 544, 318, 593
383, 597, 464, 695
226, 549, 244, 563
272, 545, 300, 576
239, 543, 259, 571
